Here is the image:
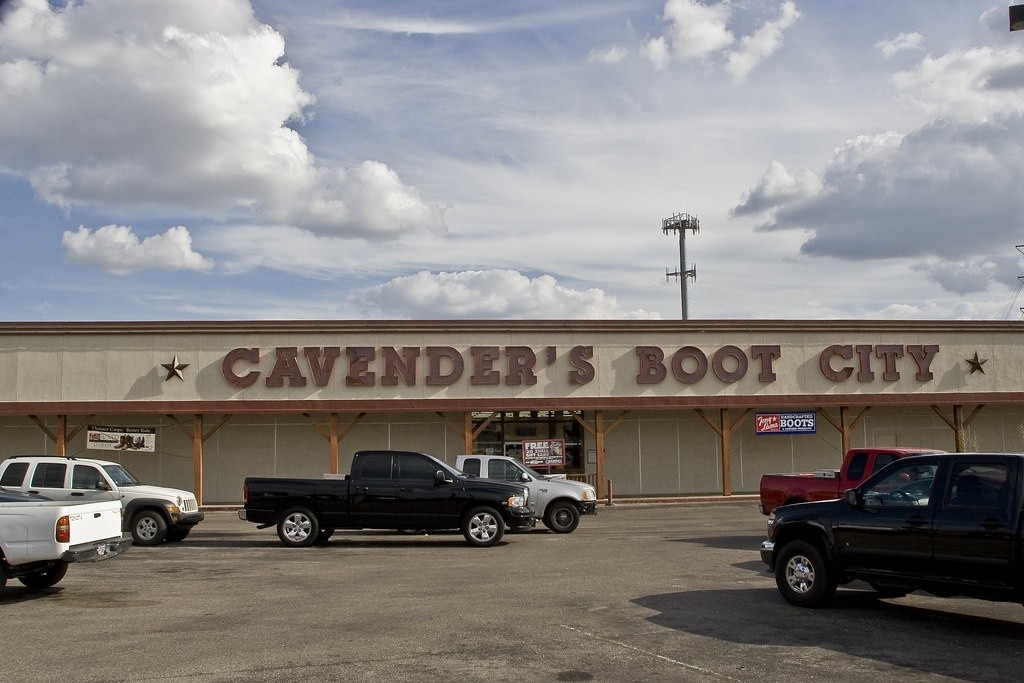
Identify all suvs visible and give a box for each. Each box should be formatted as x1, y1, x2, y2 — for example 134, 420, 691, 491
1, 455, 205, 547
763, 451, 1024, 607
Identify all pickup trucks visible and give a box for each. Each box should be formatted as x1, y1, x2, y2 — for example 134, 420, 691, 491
321, 454, 599, 534
0, 488, 136, 593
240, 448, 540, 549
759, 446, 965, 517
